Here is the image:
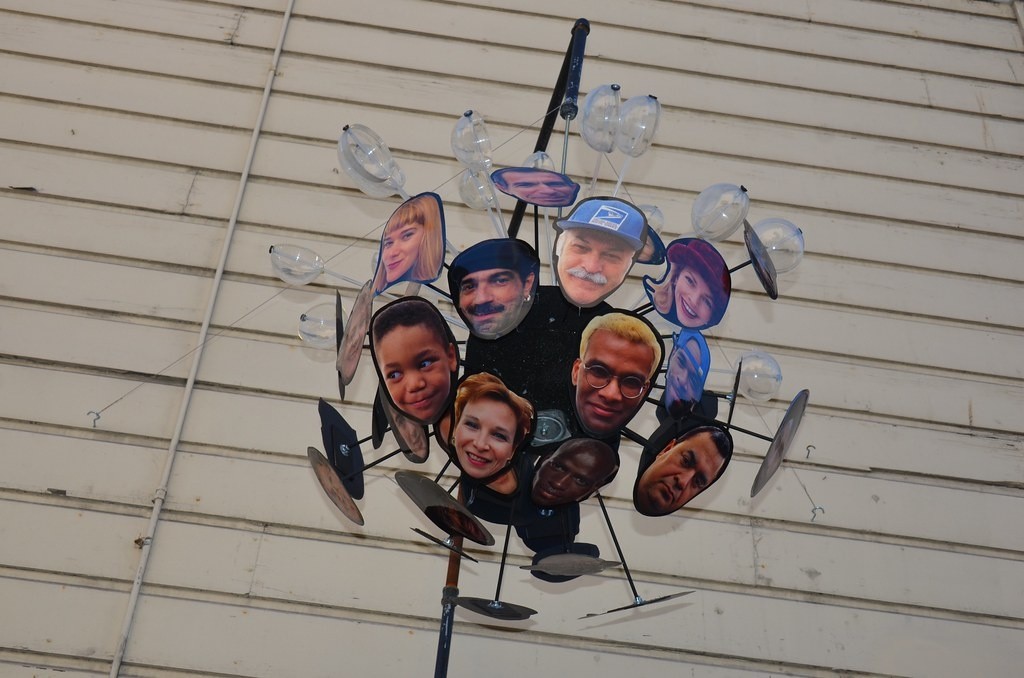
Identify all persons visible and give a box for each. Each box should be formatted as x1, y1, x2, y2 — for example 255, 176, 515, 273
533, 437, 616, 506
338, 288, 372, 379
556, 200, 644, 304
440, 416, 516, 494
316, 464, 361, 522
371, 194, 443, 296
652, 239, 730, 328
638, 428, 731, 516
760, 419, 792, 490
666, 329, 710, 419
637, 235, 655, 262
447, 236, 540, 340
452, 375, 533, 479
371, 301, 457, 420
386, 402, 427, 458
572, 313, 661, 431
442, 510, 487, 546
493, 168, 577, 206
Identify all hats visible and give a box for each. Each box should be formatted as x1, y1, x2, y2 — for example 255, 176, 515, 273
450, 238, 539, 289
676, 327, 710, 390
667, 240, 729, 309
553, 196, 646, 252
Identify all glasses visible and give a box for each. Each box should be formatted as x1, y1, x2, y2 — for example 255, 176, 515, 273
583, 362, 647, 399
